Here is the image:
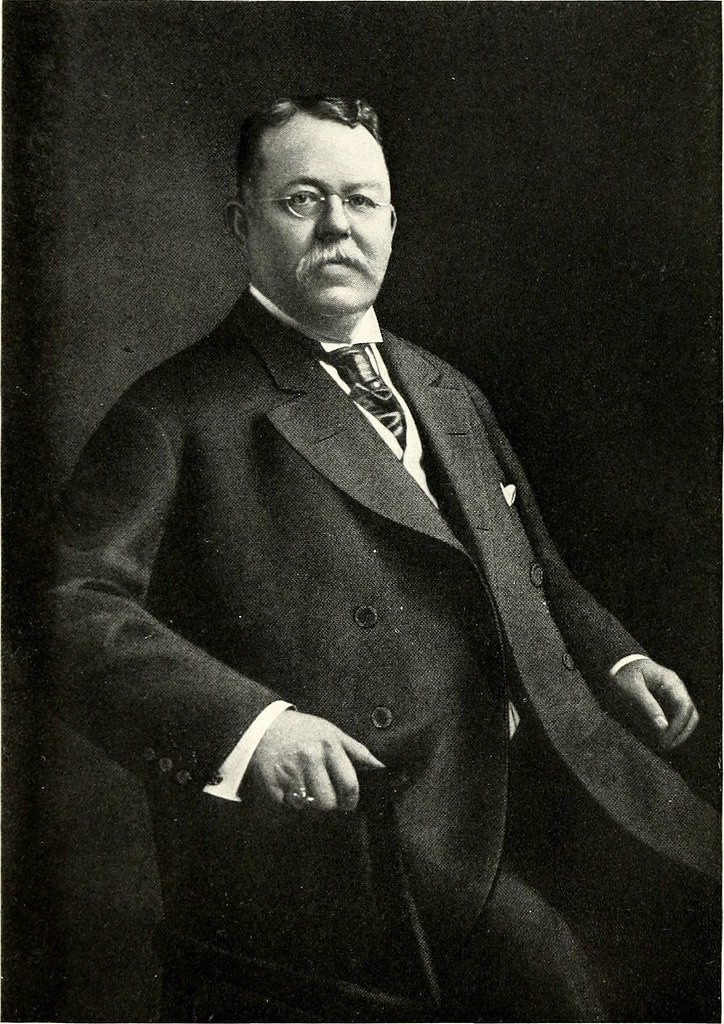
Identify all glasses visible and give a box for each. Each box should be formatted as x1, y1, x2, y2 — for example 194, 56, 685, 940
241, 190, 392, 218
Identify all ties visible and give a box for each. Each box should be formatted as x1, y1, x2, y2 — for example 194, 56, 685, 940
305, 335, 405, 446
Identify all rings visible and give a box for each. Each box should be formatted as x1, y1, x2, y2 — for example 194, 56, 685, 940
291, 788, 309, 802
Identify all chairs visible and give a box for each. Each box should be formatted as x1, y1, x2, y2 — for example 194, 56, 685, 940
145, 699, 722, 1023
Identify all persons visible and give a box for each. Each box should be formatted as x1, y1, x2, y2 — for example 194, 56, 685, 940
39, 89, 723, 1024
